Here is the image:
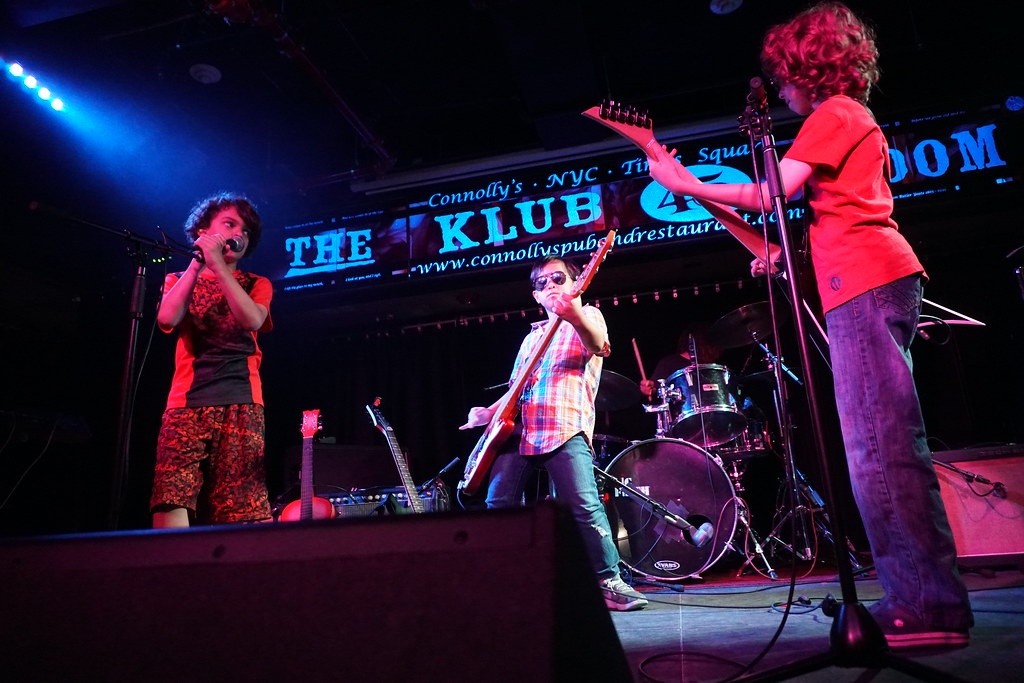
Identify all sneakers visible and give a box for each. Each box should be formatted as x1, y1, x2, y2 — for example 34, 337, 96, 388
598, 574, 648, 611
865, 593, 970, 647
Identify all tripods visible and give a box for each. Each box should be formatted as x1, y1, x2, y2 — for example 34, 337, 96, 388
728, 77, 970, 683
735, 333, 871, 581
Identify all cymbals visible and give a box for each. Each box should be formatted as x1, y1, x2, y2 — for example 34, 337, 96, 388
709, 300, 788, 347
743, 365, 802, 378
591, 433, 631, 476
596, 368, 644, 411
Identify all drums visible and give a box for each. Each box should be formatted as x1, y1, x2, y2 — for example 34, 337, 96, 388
710, 418, 772, 451
654, 363, 750, 449
597, 439, 738, 582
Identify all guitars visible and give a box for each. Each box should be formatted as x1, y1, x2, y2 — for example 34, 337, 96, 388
364, 402, 425, 514
462, 229, 617, 499
582, 97, 985, 371
277, 409, 336, 522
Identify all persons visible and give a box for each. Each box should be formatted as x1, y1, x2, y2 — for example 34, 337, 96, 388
647, 7, 974, 647
148, 194, 273, 530
458, 255, 647, 612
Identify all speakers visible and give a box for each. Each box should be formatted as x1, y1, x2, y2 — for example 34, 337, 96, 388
0, 499, 632, 683
928, 443, 1024, 570
321, 486, 435, 517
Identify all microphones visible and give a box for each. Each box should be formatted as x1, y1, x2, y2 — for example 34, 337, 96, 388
993, 482, 1008, 497
195, 237, 247, 261
688, 334, 697, 362
689, 523, 715, 548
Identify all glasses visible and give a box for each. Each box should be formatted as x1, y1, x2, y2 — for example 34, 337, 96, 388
771, 75, 783, 93
531, 271, 566, 291
